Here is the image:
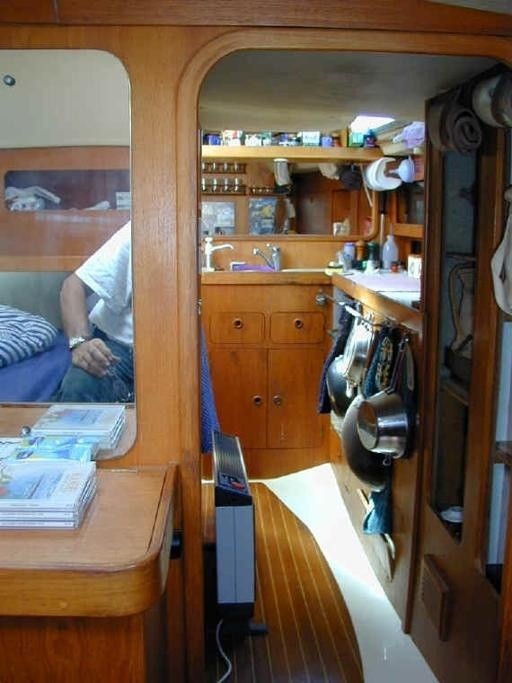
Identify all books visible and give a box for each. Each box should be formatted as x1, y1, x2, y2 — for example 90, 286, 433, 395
31, 403, 128, 450
0, 436, 99, 531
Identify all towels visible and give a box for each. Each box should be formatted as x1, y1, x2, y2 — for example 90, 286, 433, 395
197, 304, 226, 454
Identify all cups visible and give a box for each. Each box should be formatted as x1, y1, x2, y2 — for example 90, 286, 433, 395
201, 161, 275, 193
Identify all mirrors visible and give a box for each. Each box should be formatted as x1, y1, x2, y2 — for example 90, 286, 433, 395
1, 45, 140, 465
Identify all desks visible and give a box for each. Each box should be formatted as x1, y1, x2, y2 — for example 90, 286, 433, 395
1, 468, 185, 683
0, 405, 138, 467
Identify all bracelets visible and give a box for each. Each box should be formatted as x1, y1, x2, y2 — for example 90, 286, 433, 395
65, 333, 94, 352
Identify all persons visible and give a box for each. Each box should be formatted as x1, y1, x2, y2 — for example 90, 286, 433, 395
59, 220, 135, 405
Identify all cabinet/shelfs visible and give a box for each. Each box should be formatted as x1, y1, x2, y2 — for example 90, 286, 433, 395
198, 122, 425, 239
410, 61, 512, 681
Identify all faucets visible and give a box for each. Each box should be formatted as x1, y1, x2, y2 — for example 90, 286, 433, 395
251, 242, 282, 271
200, 236, 235, 273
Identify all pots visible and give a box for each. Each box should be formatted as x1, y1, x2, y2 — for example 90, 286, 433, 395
322, 307, 394, 487
353, 332, 412, 465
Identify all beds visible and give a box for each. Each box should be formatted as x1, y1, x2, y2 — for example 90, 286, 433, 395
1, 211, 130, 405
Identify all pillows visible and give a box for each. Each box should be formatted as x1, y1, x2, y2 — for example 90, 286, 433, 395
1, 269, 93, 331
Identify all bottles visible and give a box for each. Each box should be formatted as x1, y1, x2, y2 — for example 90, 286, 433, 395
342, 222, 400, 275
